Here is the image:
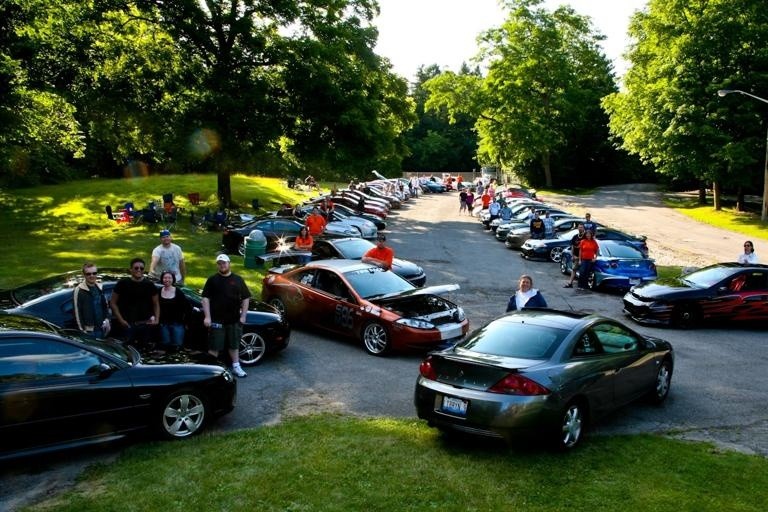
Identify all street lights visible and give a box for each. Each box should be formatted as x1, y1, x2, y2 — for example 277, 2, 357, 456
716, 90, 767, 223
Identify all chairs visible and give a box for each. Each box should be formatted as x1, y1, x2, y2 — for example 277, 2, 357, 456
106, 192, 263, 234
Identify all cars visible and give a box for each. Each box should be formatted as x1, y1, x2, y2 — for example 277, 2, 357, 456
413, 310, 675, 452
273, 235, 427, 289
262, 258, 470, 357
0, 313, 238, 465
1, 268, 291, 367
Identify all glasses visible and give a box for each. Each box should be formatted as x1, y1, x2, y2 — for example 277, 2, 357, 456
135, 266, 144, 271
83, 272, 97, 275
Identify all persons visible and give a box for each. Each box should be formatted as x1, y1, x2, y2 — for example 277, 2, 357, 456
198, 208, 210, 226
71, 264, 113, 339
148, 229, 186, 286
506, 275, 548, 311
201, 254, 251, 378
216, 207, 226, 221
738, 242, 759, 264
276, 173, 599, 290
110, 258, 160, 353
158, 271, 188, 345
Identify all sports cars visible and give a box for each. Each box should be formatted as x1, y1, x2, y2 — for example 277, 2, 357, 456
623, 264, 768, 329
521, 225, 648, 264
561, 238, 659, 291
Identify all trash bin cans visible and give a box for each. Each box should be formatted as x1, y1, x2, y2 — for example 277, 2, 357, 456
288, 175, 295, 187
252, 200, 258, 209
354, 178, 359, 184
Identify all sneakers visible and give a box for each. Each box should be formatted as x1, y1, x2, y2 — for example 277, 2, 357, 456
231, 365, 247, 377
563, 283, 585, 290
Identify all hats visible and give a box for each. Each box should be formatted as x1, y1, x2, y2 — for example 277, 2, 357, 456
216, 254, 231, 262
159, 229, 170, 237
